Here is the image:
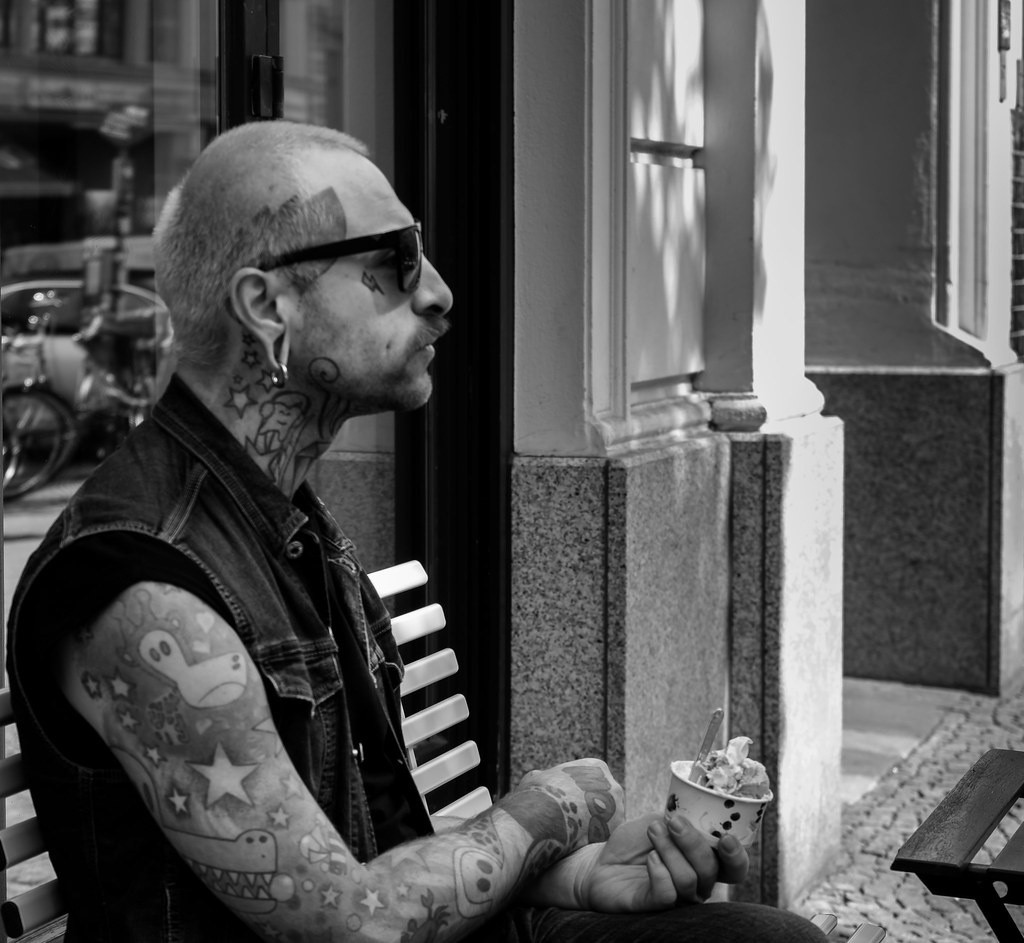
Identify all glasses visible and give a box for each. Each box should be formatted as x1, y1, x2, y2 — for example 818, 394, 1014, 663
224, 219, 424, 325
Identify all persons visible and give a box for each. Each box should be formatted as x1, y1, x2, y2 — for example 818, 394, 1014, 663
9, 122, 834, 943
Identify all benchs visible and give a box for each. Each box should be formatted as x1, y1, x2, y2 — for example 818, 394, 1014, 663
0, 557, 884, 943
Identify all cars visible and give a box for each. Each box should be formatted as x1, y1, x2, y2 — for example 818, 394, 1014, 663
0, 282, 176, 406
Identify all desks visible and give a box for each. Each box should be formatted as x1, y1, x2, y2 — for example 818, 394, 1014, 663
890, 746, 1024, 943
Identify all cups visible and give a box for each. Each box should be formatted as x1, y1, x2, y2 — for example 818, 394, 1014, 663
666, 760, 773, 846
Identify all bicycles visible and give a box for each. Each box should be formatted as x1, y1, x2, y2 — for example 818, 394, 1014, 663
1, 298, 159, 503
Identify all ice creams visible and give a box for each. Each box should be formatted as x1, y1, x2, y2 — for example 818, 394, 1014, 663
670, 708, 774, 849
